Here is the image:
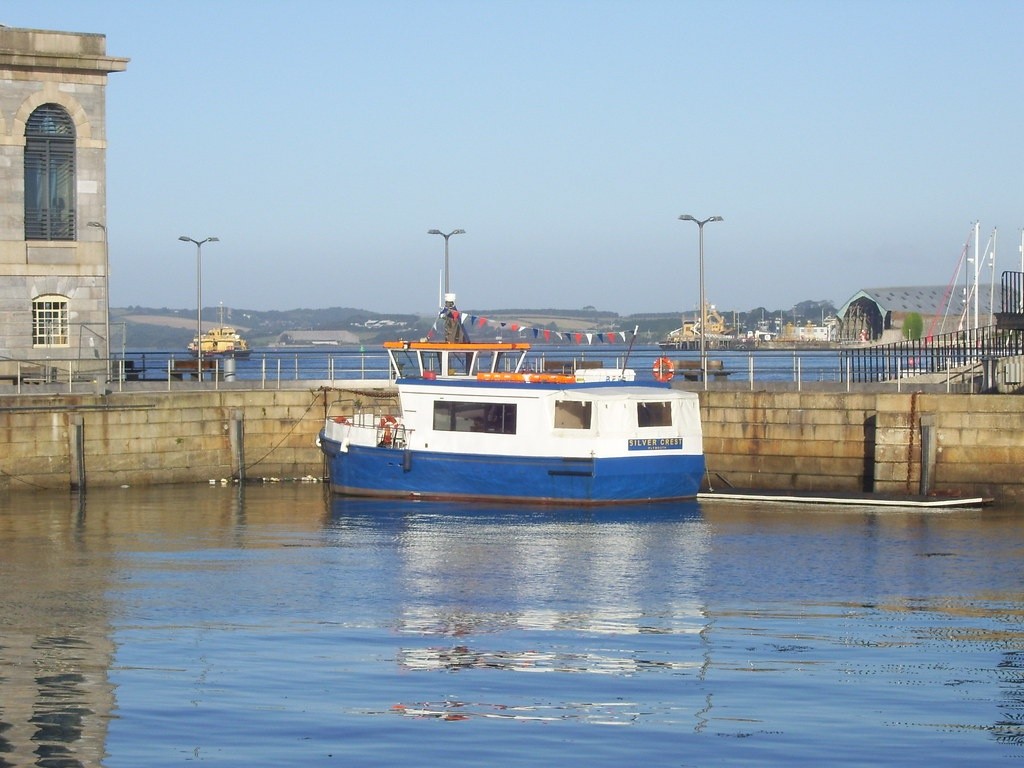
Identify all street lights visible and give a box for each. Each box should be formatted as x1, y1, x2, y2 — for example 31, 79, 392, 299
178, 236, 220, 383
679, 213, 725, 383
86, 221, 113, 383
427, 228, 466, 342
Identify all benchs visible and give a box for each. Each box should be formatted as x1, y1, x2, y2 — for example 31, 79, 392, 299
545, 360, 603, 374
672, 361, 737, 381
163, 360, 224, 381
113, 361, 147, 380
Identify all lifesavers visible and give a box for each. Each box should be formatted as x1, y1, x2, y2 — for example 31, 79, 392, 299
378, 416, 399, 445
335, 417, 351, 427
653, 358, 674, 382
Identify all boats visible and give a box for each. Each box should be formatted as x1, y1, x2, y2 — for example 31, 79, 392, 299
187, 326, 254, 360
655, 301, 760, 351
311, 270, 706, 502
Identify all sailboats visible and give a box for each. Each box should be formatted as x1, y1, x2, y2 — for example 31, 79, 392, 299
899, 219, 1024, 380
